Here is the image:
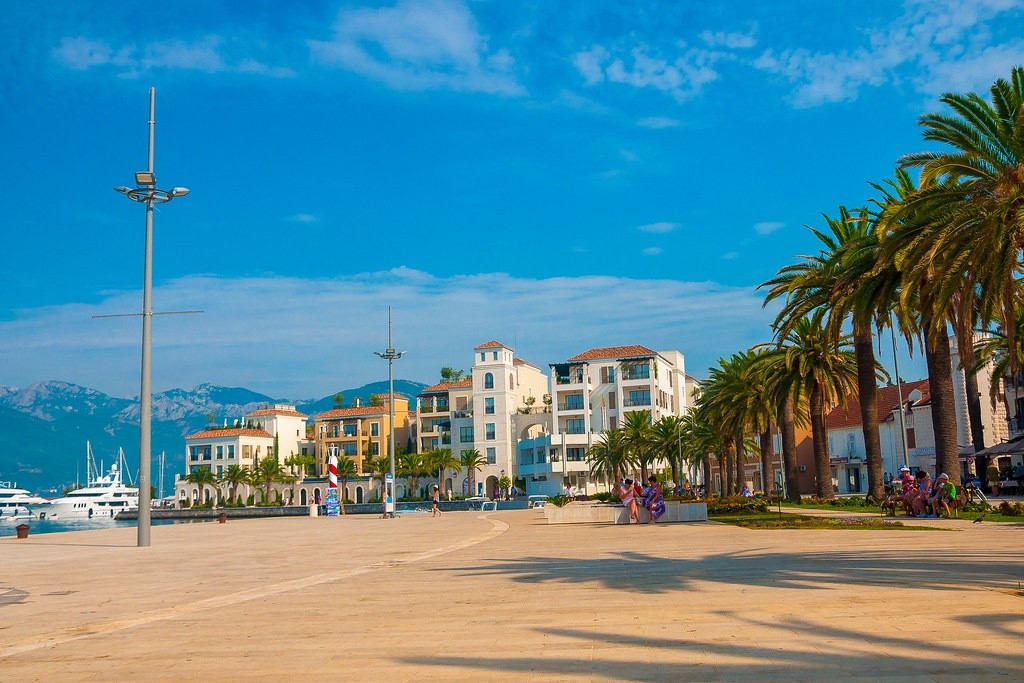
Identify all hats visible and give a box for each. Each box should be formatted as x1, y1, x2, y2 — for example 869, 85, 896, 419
908, 476, 914, 480
939, 473, 949, 479
898, 464, 910, 472
433, 485, 438, 488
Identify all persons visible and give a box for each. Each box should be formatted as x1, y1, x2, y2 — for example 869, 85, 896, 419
494, 487, 518, 500
883, 467, 956, 518
285, 494, 322, 506
1014, 462, 1024, 495
612, 475, 754, 525
985, 461, 1000, 498
565, 482, 576, 502
429, 485, 442, 517
447, 488, 452, 501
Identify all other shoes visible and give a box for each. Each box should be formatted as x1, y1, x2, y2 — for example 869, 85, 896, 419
917, 514, 927, 518
927, 514, 937, 518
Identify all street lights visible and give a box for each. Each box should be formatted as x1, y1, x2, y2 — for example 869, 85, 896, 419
113, 86, 191, 546
371, 304, 410, 520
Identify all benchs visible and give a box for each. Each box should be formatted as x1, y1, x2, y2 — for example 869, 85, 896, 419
998, 481, 1024, 497
946, 485, 968, 516
547, 506, 615, 524
612, 502, 707, 525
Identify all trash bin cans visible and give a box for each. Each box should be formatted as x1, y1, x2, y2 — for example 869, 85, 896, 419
892, 481, 903, 495
310, 503, 318, 517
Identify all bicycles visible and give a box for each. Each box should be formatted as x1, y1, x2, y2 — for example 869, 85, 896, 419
963, 476, 988, 504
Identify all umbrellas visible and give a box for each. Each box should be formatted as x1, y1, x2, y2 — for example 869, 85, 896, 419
958, 441, 1024, 463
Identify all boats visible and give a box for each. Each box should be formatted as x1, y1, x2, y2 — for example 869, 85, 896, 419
0, 483, 52, 521
22, 441, 165, 522
